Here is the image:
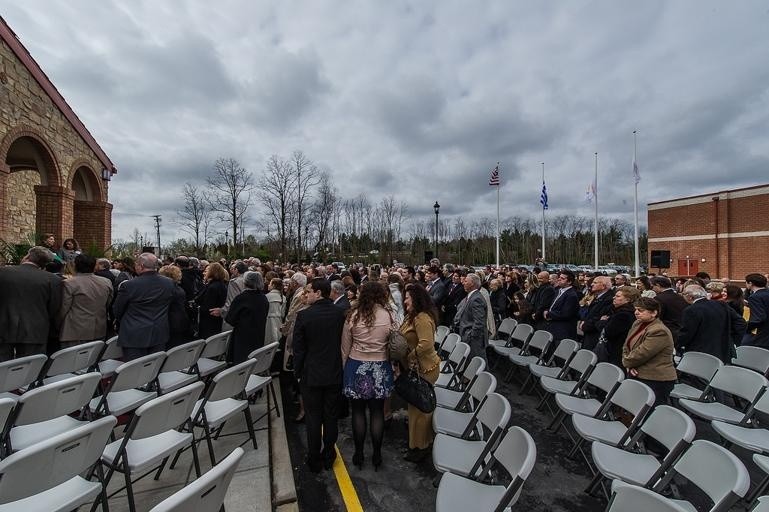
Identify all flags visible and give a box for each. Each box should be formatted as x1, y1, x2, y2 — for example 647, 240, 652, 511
489, 167, 500, 187
631, 159, 642, 187
584, 177, 597, 204
539, 180, 550, 211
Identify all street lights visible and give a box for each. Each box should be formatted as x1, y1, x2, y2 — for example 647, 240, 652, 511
139, 235, 144, 251
432, 199, 441, 260
225, 230, 229, 247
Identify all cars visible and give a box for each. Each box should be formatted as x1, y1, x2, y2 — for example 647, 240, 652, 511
470, 262, 648, 277
369, 249, 379, 255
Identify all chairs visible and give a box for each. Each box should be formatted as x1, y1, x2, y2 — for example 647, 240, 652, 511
428, 316, 769, 511
1, 328, 284, 511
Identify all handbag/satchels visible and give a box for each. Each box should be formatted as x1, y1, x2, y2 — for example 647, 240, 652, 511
395, 369, 437, 413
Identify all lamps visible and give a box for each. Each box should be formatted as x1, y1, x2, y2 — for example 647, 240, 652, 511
102, 168, 111, 181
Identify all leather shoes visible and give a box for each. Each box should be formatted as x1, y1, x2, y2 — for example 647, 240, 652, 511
305, 452, 322, 475
322, 447, 337, 473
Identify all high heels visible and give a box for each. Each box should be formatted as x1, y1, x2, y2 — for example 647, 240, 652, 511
352, 452, 364, 470
371, 455, 384, 472
